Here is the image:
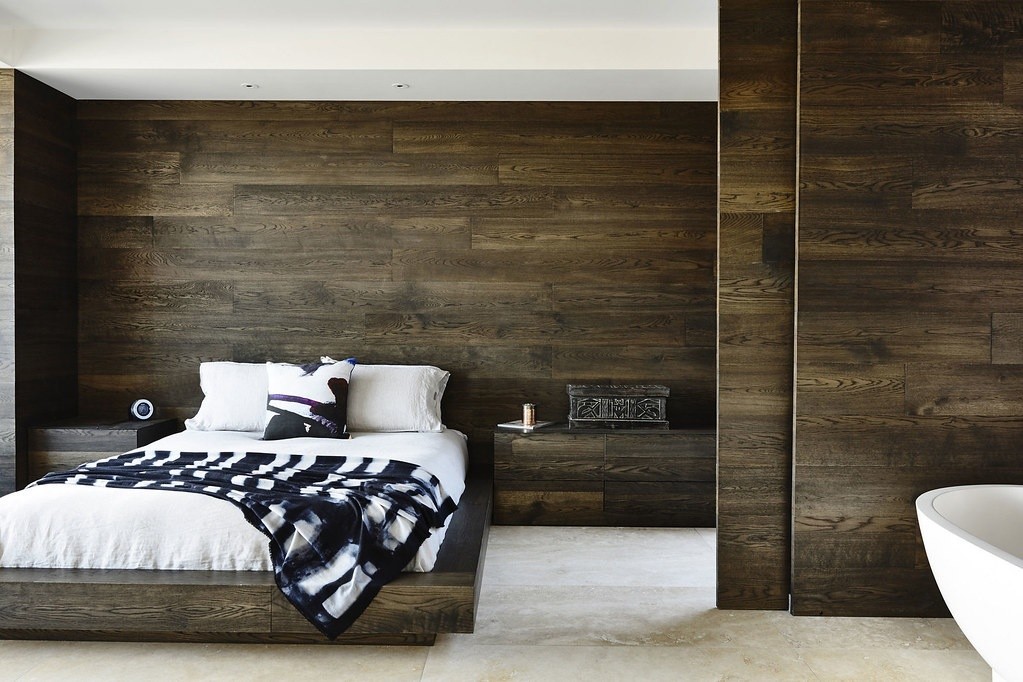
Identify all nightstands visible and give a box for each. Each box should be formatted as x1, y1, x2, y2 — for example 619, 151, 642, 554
492, 425, 717, 529
27, 417, 179, 487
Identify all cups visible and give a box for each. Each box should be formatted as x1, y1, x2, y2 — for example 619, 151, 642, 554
522, 403, 536, 425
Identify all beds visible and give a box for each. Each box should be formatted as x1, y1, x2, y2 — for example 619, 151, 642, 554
0, 429, 493, 647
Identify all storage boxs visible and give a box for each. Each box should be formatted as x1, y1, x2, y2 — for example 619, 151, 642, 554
566, 384, 670, 428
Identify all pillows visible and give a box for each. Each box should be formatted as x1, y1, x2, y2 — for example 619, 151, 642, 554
184, 361, 267, 432
259, 358, 355, 440
345, 364, 450, 433
